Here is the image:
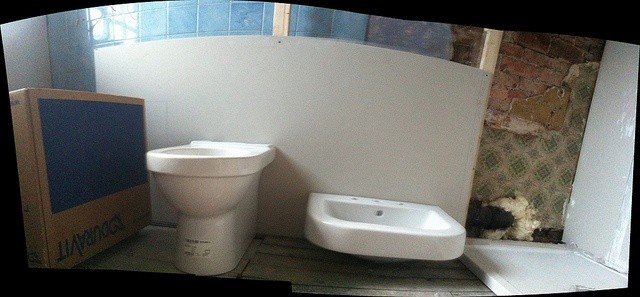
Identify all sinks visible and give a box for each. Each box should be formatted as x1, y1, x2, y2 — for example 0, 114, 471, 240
303, 193, 466, 262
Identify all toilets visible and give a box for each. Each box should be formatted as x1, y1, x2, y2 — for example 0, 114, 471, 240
146, 140, 276, 275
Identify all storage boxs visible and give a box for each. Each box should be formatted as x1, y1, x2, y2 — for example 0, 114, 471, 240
10, 86, 153, 270
